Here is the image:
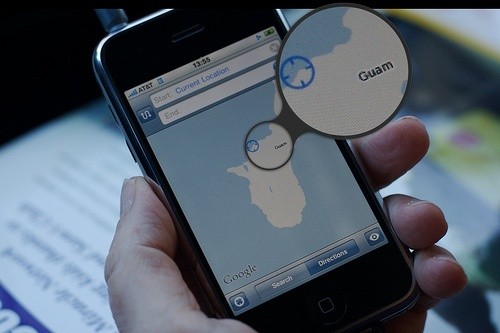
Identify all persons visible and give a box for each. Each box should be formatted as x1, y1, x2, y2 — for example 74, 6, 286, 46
104, 114, 469, 332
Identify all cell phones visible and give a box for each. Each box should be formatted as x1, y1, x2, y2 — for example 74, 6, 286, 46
93, 4, 421, 332
